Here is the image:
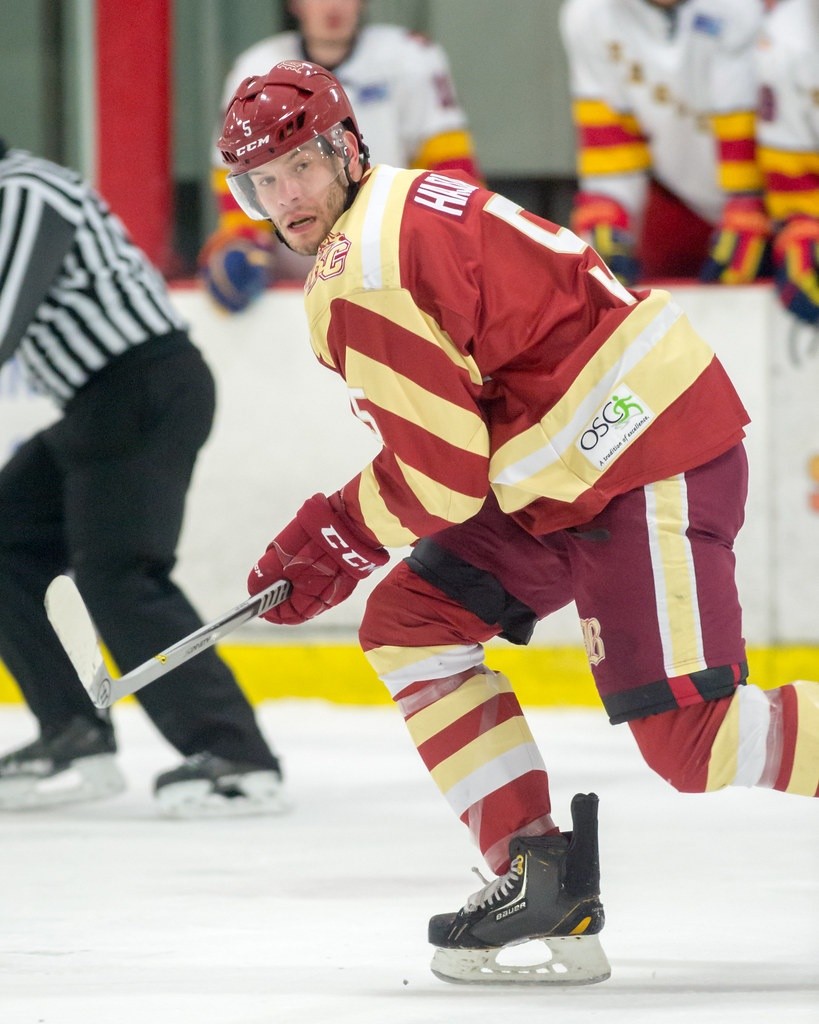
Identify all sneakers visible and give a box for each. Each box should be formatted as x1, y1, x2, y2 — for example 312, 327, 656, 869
426, 793, 611, 984
154, 739, 292, 819
0, 709, 126, 814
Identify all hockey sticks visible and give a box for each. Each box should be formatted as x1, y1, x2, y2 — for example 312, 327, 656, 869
44, 575, 292, 710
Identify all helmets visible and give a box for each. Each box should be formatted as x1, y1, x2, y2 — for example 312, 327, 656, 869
218, 59, 364, 179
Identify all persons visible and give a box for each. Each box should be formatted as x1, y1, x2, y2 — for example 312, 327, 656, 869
194, 1, 486, 311
214, 61, 818, 986
0, 126, 290, 817
554, 0, 819, 325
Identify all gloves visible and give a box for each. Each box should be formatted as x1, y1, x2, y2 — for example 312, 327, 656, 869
203, 226, 272, 312
772, 218, 819, 329
573, 221, 645, 293
246, 491, 389, 625
712, 200, 769, 288
